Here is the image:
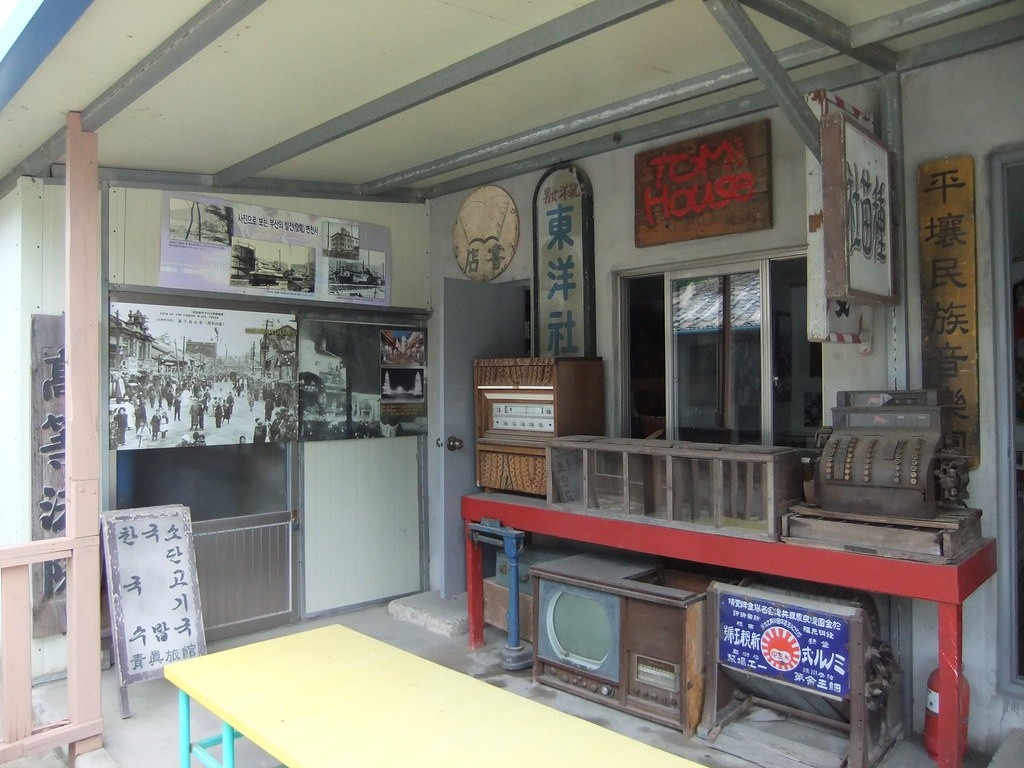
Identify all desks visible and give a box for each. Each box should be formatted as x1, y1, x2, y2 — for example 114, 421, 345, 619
461, 490, 999, 768
163, 623, 710, 768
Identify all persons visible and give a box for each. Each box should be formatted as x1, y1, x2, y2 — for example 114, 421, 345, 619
109, 360, 297, 451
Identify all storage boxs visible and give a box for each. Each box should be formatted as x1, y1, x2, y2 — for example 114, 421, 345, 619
481, 545, 576, 643
